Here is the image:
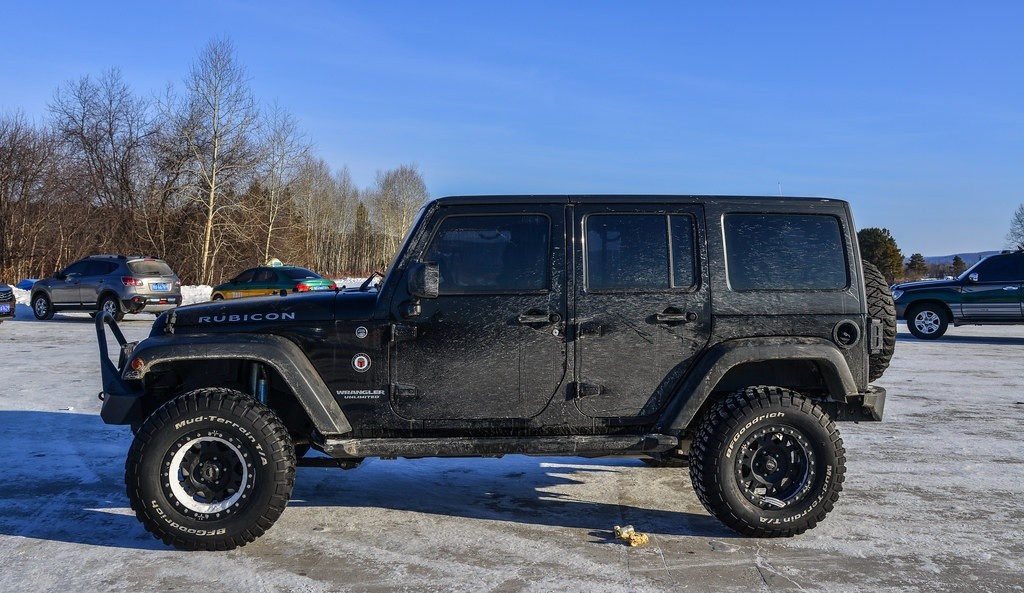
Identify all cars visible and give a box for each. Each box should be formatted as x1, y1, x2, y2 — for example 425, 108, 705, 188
210, 257, 337, 302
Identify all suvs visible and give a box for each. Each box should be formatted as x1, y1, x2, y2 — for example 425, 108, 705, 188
30, 253, 183, 323
94, 194, 898, 553
889, 244, 1024, 340
